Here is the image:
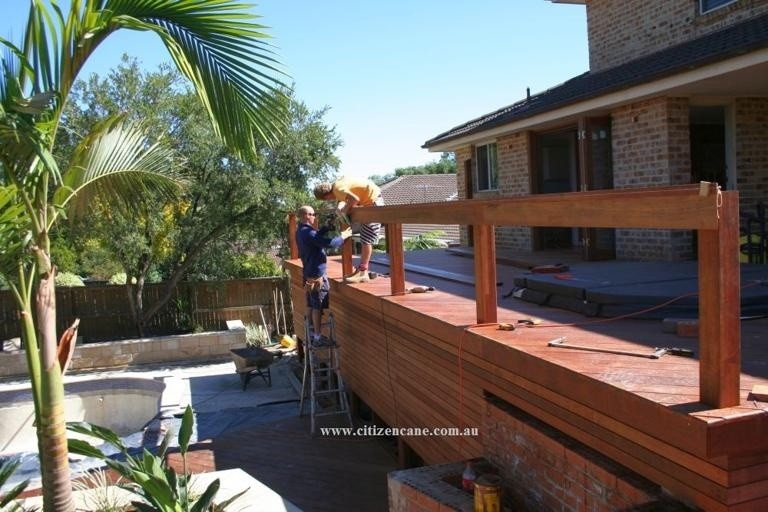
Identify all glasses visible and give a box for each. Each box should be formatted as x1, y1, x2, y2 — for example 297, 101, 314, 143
308, 213, 316, 217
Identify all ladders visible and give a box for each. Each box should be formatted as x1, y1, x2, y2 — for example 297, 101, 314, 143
297, 313, 354, 434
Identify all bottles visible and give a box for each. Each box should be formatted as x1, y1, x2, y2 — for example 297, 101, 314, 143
460, 460, 477, 491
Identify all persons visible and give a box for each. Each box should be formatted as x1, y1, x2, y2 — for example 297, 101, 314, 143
313, 175, 385, 284
294, 204, 354, 348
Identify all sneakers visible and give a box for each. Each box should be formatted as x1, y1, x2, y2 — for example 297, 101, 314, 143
309, 334, 334, 348
344, 267, 370, 284
